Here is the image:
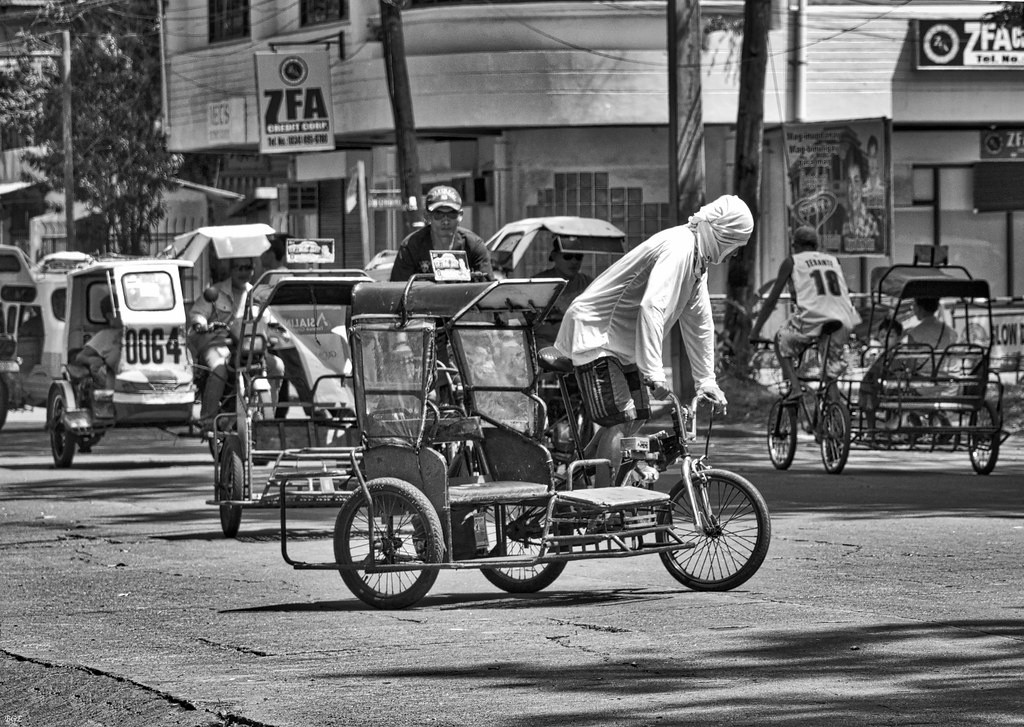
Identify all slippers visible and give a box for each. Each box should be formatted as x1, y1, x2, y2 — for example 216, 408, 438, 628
781, 397, 798, 405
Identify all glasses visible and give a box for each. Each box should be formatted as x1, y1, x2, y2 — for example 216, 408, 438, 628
430, 210, 458, 220
557, 251, 583, 261
233, 263, 253, 272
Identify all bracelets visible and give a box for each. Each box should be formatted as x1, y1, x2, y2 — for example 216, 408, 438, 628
501, 335, 515, 340
393, 341, 410, 349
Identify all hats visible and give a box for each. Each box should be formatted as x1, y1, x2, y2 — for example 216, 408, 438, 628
426, 185, 462, 210
791, 226, 819, 247
549, 233, 584, 262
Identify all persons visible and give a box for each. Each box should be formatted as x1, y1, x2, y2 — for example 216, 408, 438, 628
188, 257, 291, 436
906, 295, 959, 443
553, 195, 754, 535
532, 238, 595, 443
841, 147, 879, 252
865, 319, 905, 440
76, 293, 124, 391
389, 186, 525, 381
750, 227, 861, 430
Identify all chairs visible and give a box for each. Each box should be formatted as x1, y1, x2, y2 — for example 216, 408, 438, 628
879, 341, 983, 412
371, 403, 556, 564
247, 374, 359, 499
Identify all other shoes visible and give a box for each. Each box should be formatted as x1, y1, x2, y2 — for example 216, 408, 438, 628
932, 411, 953, 444
586, 512, 632, 533
904, 413, 923, 440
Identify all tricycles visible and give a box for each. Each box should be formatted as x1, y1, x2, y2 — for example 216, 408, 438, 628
748, 246, 1010, 475
44, 223, 273, 470
206, 268, 474, 537
281, 270, 772, 609
